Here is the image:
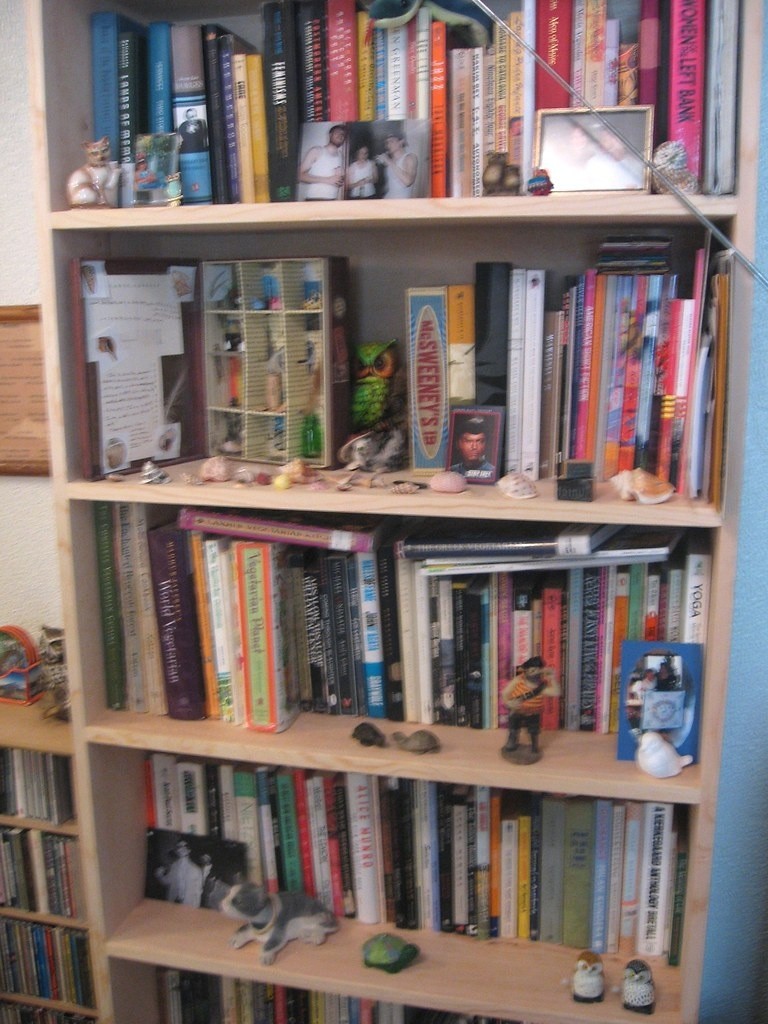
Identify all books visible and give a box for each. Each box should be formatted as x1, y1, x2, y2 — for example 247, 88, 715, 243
152, 963, 520, 1024
141, 751, 690, 967
0, 914, 98, 1007
469, 239, 732, 519
1, 998, 95, 1024
1, 824, 87, 921
95, 495, 710, 739
89, 1, 739, 199
0, 742, 72, 823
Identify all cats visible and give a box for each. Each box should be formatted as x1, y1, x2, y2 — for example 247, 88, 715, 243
219, 874, 340, 967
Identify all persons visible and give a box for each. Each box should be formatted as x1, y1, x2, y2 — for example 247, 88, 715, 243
135, 155, 155, 206
192, 851, 220, 911
630, 667, 645, 683
628, 666, 657, 703
499, 656, 560, 755
177, 106, 208, 153
543, 120, 611, 192
451, 416, 493, 478
379, 131, 420, 199
653, 661, 678, 691
153, 837, 204, 908
347, 144, 379, 199
296, 123, 351, 200
591, 122, 644, 189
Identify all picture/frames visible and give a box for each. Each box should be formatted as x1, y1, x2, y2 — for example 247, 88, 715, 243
617, 639, 705, 766
527, 104, 656, 197
444, 404, 506, 487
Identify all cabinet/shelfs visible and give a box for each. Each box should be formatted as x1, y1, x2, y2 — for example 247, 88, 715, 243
0, 687, 103, 1024
24, 0, 762, 1024
